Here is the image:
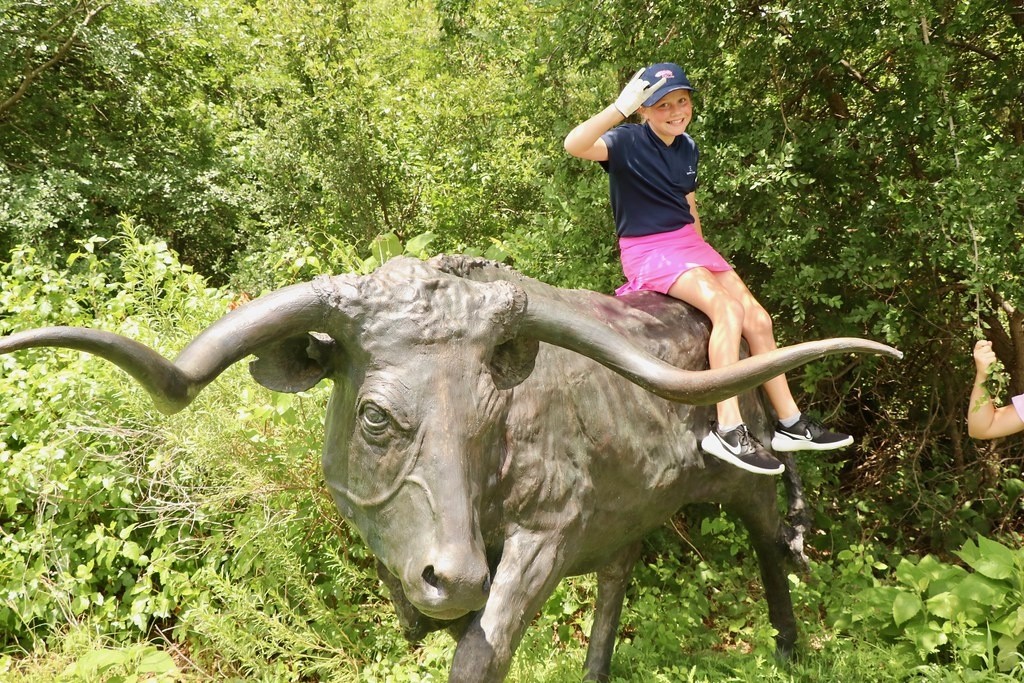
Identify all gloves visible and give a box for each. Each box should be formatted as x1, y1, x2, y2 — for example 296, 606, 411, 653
614, 67, 666, 118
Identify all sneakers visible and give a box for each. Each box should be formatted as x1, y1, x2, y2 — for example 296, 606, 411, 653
771, 412, 854, 451
701, 424, 786, 475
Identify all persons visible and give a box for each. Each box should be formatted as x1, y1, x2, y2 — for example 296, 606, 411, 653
563, 62, 855, 475
968, 339, 1024, 439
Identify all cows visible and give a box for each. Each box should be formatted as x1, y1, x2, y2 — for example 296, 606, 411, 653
0, 252, 904, 683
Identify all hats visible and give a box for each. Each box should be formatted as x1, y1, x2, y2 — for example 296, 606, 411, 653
638, 62, 697, 107
